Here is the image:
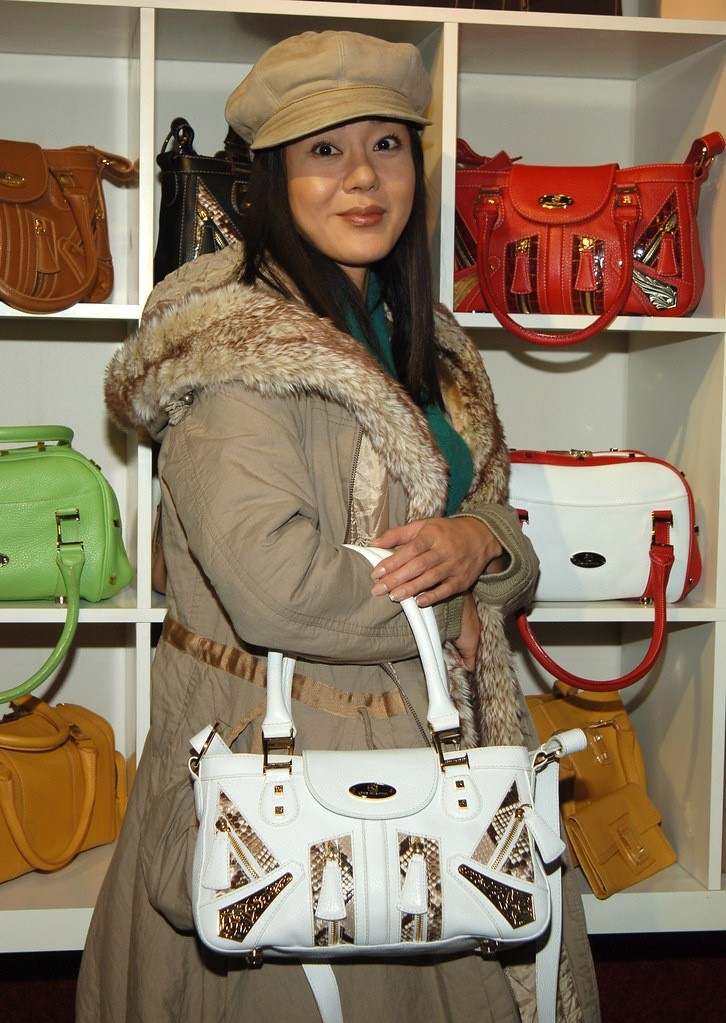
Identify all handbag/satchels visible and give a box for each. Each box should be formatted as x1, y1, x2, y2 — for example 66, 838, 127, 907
505, 448, 703, 693
0, 139, 135, 315
0, 693, 127, 884
0, 424, 132, 704
152, 116, 254, 287
186, 542, 588, 1023
347, 0, 623, 17
523, 679, 678, 901
454, 130, 725, 347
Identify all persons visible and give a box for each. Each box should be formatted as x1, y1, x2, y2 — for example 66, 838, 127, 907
74, 30, 601, 1023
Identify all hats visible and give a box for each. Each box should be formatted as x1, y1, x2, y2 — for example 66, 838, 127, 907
224, 29, 434, 161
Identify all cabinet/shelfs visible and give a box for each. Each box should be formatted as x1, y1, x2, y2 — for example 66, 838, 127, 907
0, 0, 726, 953
